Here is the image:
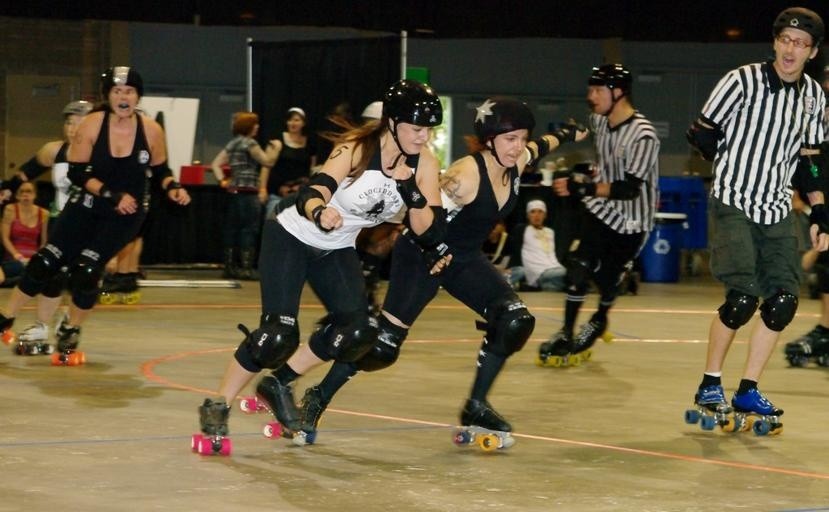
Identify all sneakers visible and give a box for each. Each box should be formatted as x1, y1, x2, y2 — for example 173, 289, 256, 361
223, 267, 240, 279
242, 266, 259, 280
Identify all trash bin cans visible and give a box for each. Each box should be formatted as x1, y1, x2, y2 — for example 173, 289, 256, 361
639, 212, 688, 282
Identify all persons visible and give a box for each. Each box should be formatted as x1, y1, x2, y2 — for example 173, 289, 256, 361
785, 46, 828, 358
198, 77, 442, 440
534, 60, 664, 356
507, 199, 639, 295
0, 67, 191, 366
211, 107, 316, 279
296, 92, 590, 445
686, 24, 828, 413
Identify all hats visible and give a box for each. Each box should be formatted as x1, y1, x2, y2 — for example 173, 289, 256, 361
102, 66, 143, 100
527, 200, 546, 211
63, 100, 94, 115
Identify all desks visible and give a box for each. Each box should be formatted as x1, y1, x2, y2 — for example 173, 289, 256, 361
138, 178, 577, 279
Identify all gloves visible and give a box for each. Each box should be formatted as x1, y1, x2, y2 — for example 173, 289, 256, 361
419, 233, 450, 269
557, 124, 578, 142
567, 178, 597, 200
809, 204, 828, 235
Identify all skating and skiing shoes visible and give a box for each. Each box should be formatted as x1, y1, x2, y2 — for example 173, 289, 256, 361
685, 386, 741, 432
240, 374, 303, 438
98, 272, 140, 304
192, 396, 231, 456
14, 320, 53, 355
452, 398, 516, 451
784, 325, 829, 368
281, 386, 327, 444
52, 322, 85, 366
538, 330, 573, 367
1, 313, 15, 345
572, 313, 615, 366
731, 389, 784, 436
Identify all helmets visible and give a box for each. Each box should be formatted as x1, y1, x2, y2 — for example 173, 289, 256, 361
777, 36, 810, 50
362, 101, 383, 119
384, 79, 443, 127
474, 99, 534, 144
589, 63, 632, 88
289, 107, 305, 117
774, 7, 824, 45
